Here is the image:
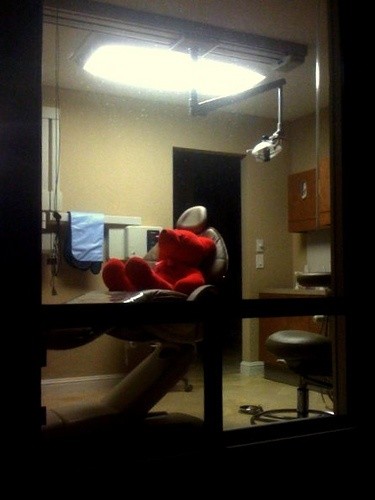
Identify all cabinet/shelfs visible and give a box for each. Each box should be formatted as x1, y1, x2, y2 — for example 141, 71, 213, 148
257, 292, 308, 387
287, 168, 318, 232
317, 152, 332, 228
308, 296, 329, 393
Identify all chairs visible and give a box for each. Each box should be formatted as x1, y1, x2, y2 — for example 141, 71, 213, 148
251, 272, 333, 424
47, 205, 229, 433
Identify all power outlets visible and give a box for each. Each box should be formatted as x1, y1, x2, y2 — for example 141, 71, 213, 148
256, 255, 264, 268
